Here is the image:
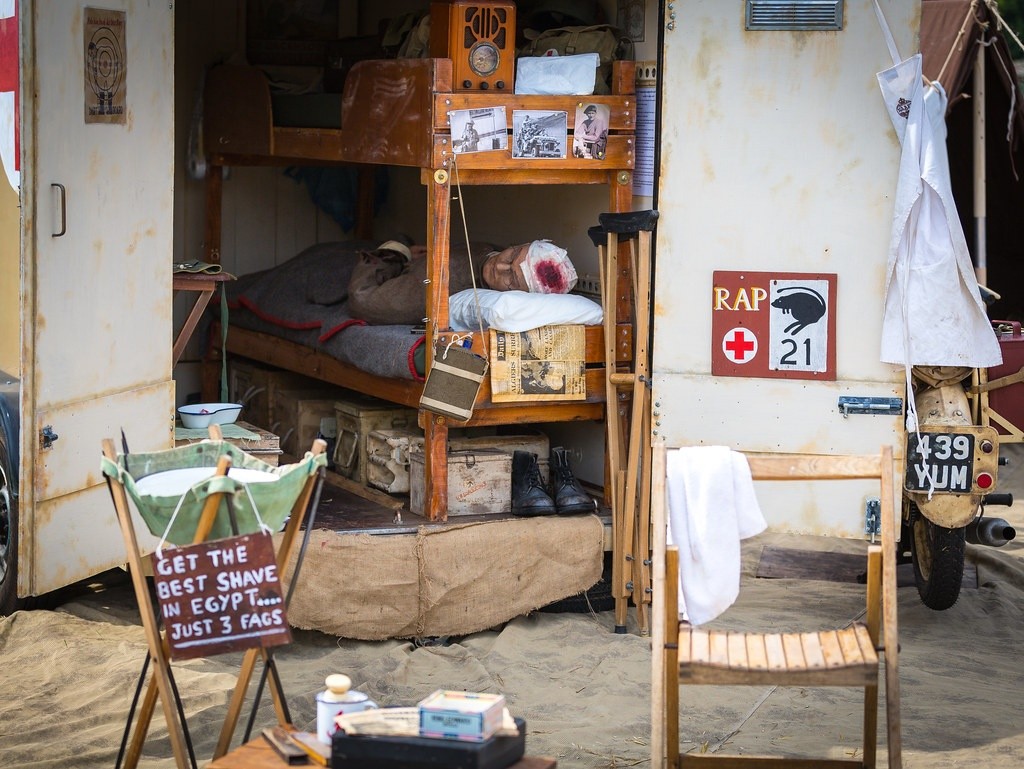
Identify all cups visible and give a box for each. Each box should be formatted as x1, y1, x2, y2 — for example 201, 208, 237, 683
316, 690, 378, 745
613, 60, 635, 95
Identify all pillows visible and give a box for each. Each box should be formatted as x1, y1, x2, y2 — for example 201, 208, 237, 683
448, 288, 604, 329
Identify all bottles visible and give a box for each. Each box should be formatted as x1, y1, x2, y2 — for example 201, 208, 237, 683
450, 335, 482, 358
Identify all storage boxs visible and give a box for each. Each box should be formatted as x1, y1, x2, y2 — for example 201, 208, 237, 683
230, 362, 337, 433
367, 426, 496, 495
418, 688, 505, 741
407, 446, 514, 517
276, 388, 375, 458
330, 704, 527, 769
330, 398, 418, 487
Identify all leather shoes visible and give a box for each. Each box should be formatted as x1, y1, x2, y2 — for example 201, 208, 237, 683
510, 450, 556, 515
546, 446, 596, 528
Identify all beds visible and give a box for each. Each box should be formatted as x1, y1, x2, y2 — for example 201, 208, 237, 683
203, 54, 639, 526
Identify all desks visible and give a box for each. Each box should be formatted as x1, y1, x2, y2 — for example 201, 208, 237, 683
207, 730, 557, 769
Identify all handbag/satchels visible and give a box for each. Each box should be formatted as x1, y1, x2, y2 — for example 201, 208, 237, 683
418, 344, 489, 423
518, 24, 633, 96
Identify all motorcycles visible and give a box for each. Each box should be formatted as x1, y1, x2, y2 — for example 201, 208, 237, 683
906, 275, 1017, 609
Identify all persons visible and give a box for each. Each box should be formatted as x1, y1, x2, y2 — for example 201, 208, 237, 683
461, 120, 479, 152
288, 236, 577, 325
522, 115, 532, 128
575, 105, 603, 155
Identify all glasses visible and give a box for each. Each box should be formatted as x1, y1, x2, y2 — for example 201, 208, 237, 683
504, 243, 529, 291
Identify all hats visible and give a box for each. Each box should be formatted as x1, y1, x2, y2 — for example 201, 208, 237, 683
584, 105, 597, 114
467, 120, 475, 125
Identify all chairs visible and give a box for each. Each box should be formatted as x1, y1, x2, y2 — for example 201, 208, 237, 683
648, 434, 903, 769
